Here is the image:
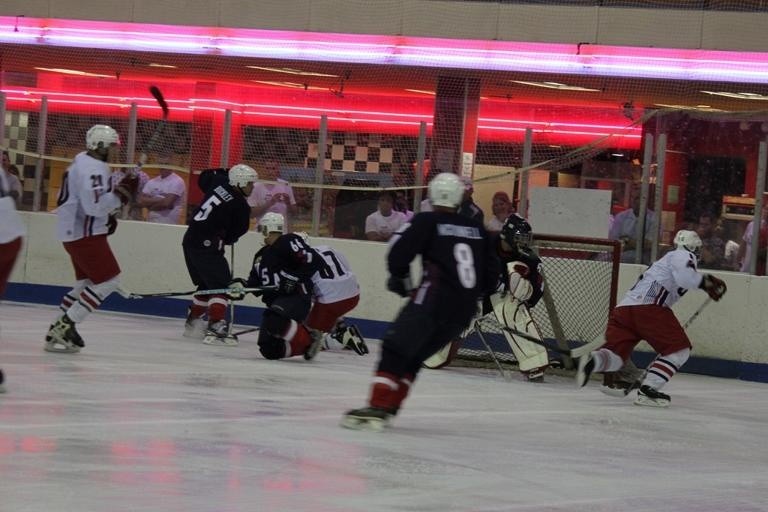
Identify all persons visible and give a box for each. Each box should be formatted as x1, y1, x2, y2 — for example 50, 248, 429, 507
228, 213, 322, 360
758, 205, 768, 275
1, 166, 26, 394
714, 225, 739, 263
337, 171, 503, 434
307, 245, 369, 354
736, 205, 765, 273
44, 124, 141, 354
457, 176, 485, 226
1, 150, 23, 200
112, 146, 149, 222
248, 155, 297, 232
136, 154, 186, 224
365, 190, 406, 242
695, 212, 725, 269
575, 229, 727, 409
607, 185, 661, 251
182, 164, 257, 346
421, 212, 547, 382
486, 191, 531, 232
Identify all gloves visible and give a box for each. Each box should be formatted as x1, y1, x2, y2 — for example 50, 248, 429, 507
105, 213, 118, 235
112, 173, 140, 206
700, 273, 727, 302
226, 277, 248, 301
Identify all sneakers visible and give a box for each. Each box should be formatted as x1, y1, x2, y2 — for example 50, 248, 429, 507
203, 319, 239, 346
45, 313, 85, 348
182, 316, 206, 339
344, 406, 398, 423
576, 352, 595, 387
304, 329, 321, 361
342, 327, 366, 355
637, 385, 671, 404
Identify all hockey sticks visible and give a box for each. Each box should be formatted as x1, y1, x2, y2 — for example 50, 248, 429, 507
116, 286, 279, 300
481, 316, 607, 358
599, 296, 711, 398
131, 84, 169, 177
477, 329, 512, 382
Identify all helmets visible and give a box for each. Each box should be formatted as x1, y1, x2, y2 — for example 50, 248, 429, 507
427, 172, 465, 211
255, 212, 284, 245
86, 124, 121, 150
293, 230, 311, 246
501, 212, 532, 250
228, 164, 258, 188
673, 230, 703, 262
